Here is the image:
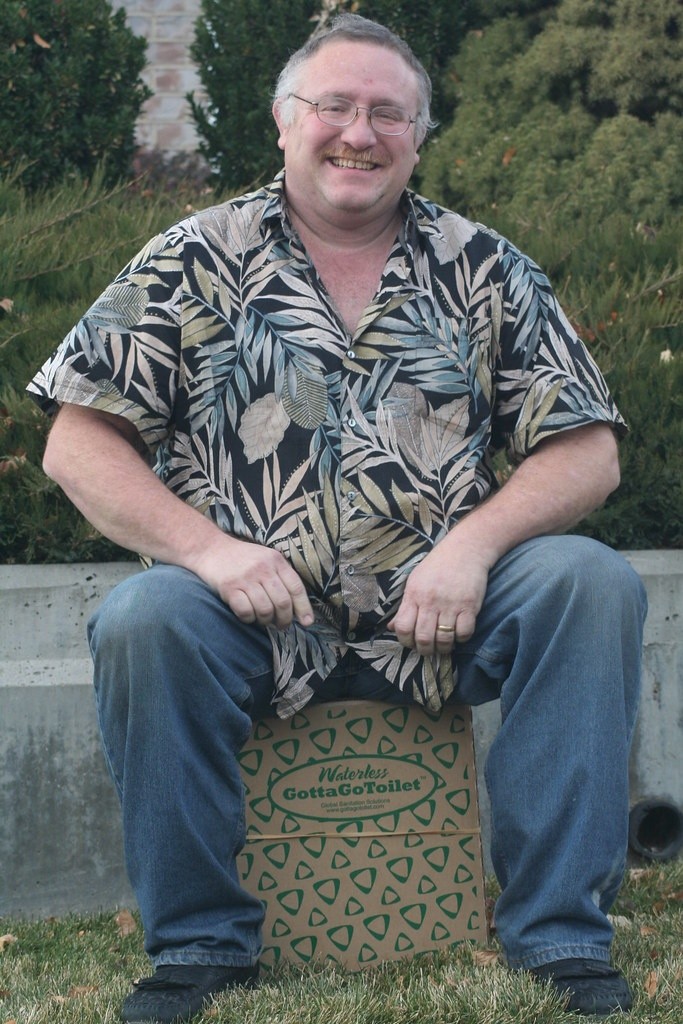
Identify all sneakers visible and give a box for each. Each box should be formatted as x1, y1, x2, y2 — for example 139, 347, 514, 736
534, 961, 635, 1013
116, 963, 259, 1022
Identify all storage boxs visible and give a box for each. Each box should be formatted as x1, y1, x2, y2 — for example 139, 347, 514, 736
236, 702, 488, 980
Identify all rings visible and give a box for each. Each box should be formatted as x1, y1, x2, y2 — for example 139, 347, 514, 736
436, 624, 455, 633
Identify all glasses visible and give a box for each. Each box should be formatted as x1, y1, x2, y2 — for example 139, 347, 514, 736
286, 93, 423, 137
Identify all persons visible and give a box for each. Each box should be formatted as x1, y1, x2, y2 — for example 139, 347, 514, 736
22, 12, 650, 1024
